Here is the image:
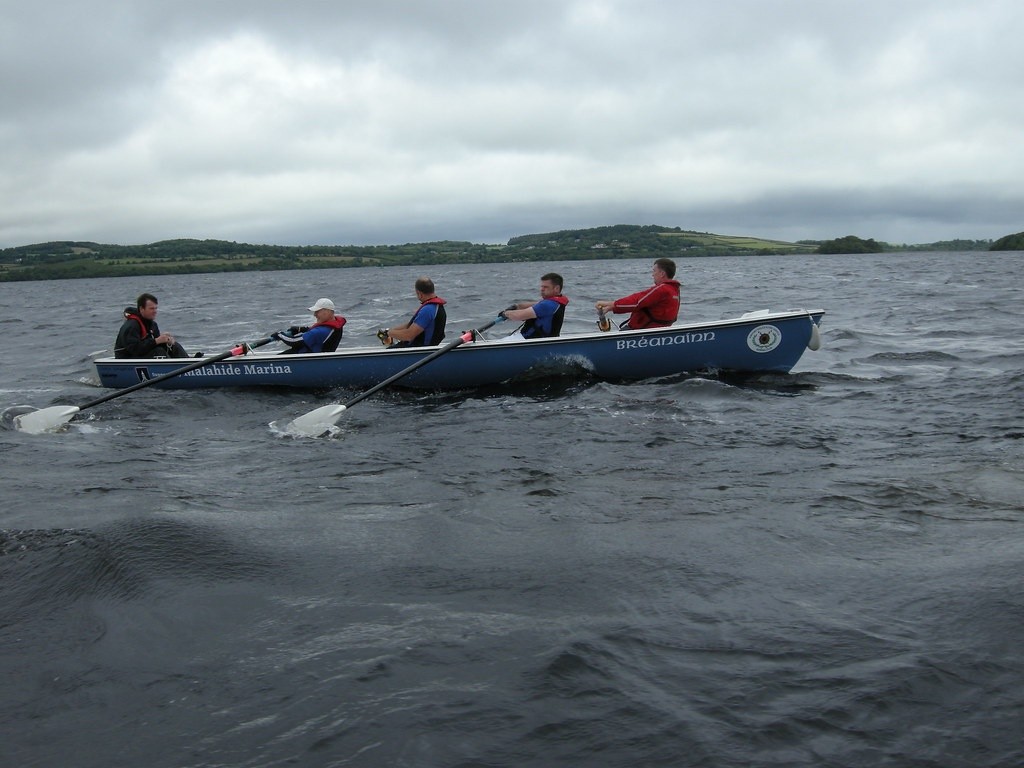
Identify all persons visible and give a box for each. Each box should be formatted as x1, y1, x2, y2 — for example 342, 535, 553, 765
498, 272, 569, 341
114, 293, 204, 359
377, 277, 447, 350
270, 297, 347, 355
595, 258, 682, 331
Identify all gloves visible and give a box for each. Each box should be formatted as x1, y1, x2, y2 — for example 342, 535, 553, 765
271, 331, 282, 341
498, 310, 508, 321
287, 326, 300, 335
506, 305, 517, 310
378, 328, 389, 340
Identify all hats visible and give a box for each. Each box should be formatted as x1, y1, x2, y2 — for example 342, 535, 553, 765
308, 298, 335, 312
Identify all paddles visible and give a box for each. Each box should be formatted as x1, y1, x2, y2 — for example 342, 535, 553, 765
287, 316, 504, 439
597, 305, 611, 332
379, 333, 400, 345
18, 331, 291, 434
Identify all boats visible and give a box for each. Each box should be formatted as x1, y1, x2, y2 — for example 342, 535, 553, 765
94, 307, 828, 392
